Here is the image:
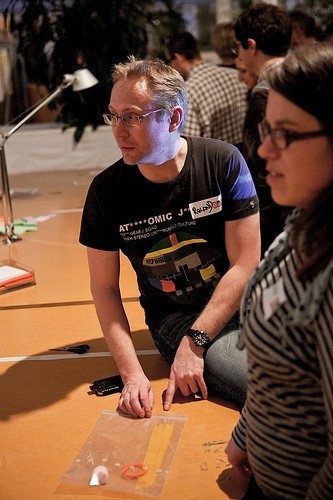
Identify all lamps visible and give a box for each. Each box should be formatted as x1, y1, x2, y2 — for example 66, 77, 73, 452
0, 69, 99, 242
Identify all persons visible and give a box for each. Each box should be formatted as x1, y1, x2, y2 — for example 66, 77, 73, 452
223, 44, 333, 500
162, 4, 323, 260
79, 55, 262, 419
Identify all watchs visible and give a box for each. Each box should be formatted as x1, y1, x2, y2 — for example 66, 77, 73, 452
186, 329, 212, 349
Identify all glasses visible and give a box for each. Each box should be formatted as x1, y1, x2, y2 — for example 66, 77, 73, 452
230, 41, 246, 55
102, 108, 163, 129
257, 115, 333, 151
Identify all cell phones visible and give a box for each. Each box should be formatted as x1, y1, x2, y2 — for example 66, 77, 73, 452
90, 375, 124, 396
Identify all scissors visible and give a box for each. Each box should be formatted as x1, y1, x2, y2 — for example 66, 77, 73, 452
49, 344, 90, 355
